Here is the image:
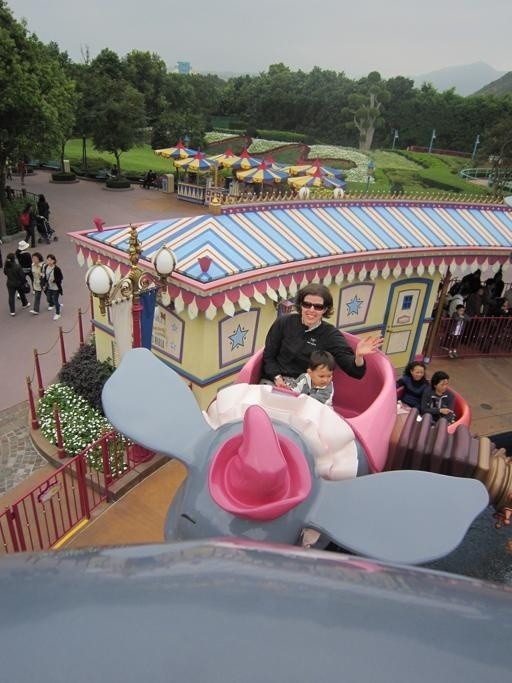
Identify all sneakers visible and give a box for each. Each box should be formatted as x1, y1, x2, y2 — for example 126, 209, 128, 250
10, 290, 64, 321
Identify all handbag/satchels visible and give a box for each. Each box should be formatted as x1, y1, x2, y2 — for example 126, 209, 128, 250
18, 283, 31, 293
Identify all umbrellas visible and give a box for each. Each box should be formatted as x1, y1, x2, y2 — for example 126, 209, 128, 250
153, 137, 348, 192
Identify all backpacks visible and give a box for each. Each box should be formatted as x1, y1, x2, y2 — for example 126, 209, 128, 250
20, 213, 29, 225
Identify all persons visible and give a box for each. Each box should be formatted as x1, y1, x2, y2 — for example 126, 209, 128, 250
396, 361, 455, 423
15, 159, 27, 185
5, 157, 14, 180
37, 194, 50, 220
22, 202, 38, 247
439, 269, 512, 359
258, 284, 385, 409
108, 163, 117, 179
4, 240, 64, 320
143, 169, 157, 190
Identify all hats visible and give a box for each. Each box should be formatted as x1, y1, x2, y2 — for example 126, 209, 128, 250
18, 241, 30, 250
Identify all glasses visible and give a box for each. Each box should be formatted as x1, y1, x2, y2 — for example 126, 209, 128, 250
301, 301, 323, 311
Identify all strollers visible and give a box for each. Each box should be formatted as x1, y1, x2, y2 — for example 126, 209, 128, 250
36, 216, 58, 245
